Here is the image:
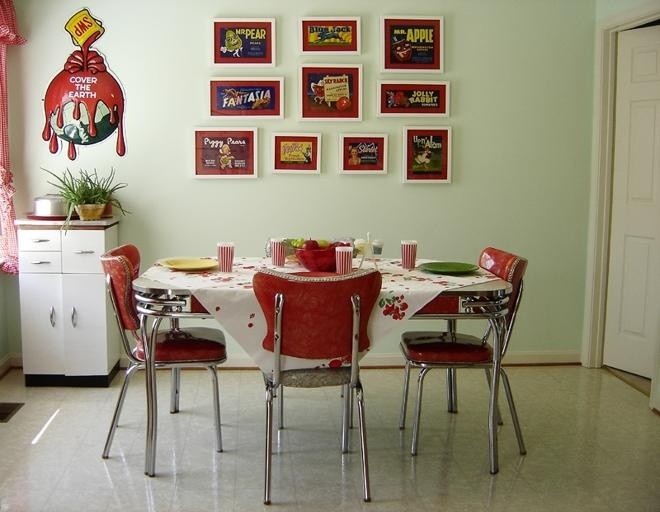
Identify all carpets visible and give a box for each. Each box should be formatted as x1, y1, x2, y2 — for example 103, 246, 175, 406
0, 402, 26, 425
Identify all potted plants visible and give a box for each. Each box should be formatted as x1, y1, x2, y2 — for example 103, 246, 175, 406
38, 164, 134, 235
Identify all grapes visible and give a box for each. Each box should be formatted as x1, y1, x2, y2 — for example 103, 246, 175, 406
291, 238, 304, 248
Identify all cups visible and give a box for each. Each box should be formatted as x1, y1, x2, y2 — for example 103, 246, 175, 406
400, 240, 417, 270
216, 242, 234, 272
270, 239, 286, 267
335, 246, 352, 274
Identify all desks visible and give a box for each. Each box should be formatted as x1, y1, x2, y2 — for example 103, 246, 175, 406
131, 256, 515, 481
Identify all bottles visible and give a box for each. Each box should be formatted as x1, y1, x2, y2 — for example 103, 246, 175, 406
373, 239, 383, 254
354, 239, 366, 255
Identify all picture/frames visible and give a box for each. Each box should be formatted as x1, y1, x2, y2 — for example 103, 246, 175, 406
206, 76, 285, 121
376, 80, 450, 118
270, 132, 322, 173
402, 125, 452, 184
298, 61, 364, 123
380, 14, 445, 74
337, 133, 389, 176
209, 17, 277, 68
191, 126, 258, 179
297, 15, 362, 55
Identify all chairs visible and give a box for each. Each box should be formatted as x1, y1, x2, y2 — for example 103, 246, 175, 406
398, 246, 528, 459
97, 242, 228, 459
248, 266, 383, 506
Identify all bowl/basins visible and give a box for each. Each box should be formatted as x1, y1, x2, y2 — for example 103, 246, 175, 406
284, 246, 297, 260
292, 242, 350, 274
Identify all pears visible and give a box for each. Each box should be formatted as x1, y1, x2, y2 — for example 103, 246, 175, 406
317, 240, 331, 247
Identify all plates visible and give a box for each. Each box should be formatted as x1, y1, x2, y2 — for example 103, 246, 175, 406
158, 257, 218, 271
419, 261, 480, 274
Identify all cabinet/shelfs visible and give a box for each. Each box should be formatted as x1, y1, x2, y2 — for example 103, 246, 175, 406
13, 215, 121, 388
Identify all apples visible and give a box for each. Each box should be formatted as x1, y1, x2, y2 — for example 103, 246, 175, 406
329, 242, 345, 248
301, 240, 318, 249
